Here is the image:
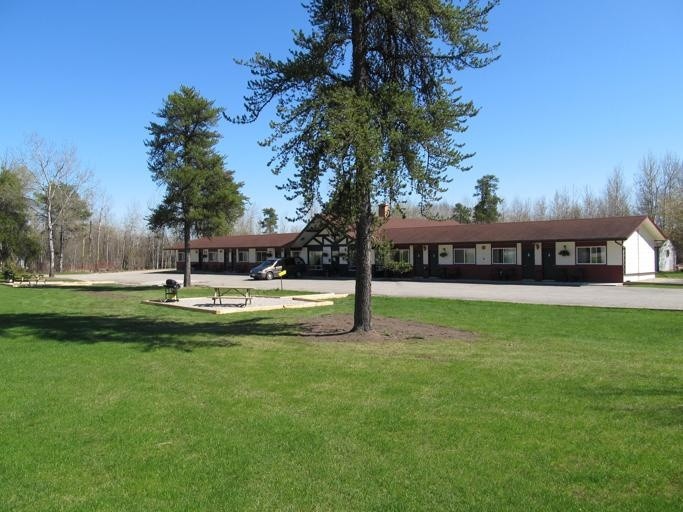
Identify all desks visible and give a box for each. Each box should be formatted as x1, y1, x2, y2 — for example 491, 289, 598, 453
206, 285, 255, 306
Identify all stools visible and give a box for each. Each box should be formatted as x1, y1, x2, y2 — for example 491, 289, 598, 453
163, 285, 179, 302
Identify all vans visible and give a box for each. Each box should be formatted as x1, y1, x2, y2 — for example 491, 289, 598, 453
248, 257, 306, 280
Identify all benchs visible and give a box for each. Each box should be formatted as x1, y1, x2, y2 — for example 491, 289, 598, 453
207, 295, 213, 298
212, 296, 253, 299
14, 275, 48, 285
